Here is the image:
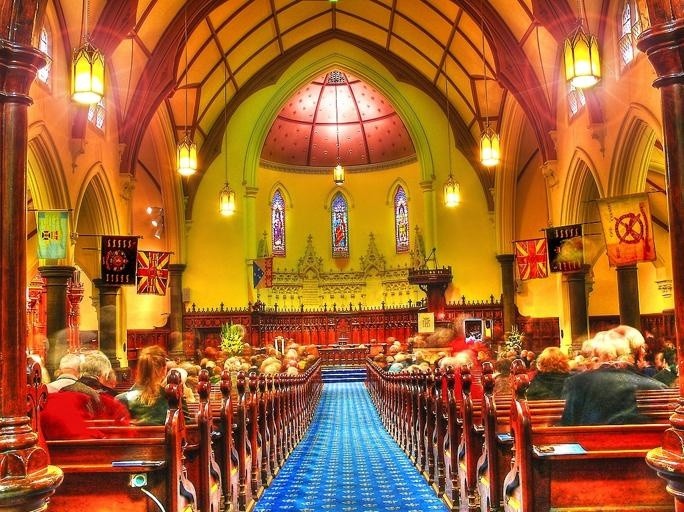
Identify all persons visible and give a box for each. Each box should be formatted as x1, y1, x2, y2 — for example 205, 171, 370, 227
367, 327, 675, 428
33, 326, 318, 440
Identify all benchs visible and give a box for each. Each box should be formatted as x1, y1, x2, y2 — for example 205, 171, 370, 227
50, 352, 321, 511
370, 355, 680, 512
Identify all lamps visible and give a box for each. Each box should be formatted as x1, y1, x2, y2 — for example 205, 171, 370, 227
442, 53, 461, 209
148, 206, 165, 240
177, 7, 199, 178
563, 0, 602, 89
69, 0, 106, 105
478, 1, 502, 168
219, 55, 237, 218
333, 79, 346, 186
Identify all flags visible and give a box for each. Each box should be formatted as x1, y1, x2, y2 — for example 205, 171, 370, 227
252, 258, 272, 288
35, 210, 68, 259
515, 239, 548, 280
596, 192, 656, 268
545, 223, 584, 273
100, 235, 138, 285
417, 312, 434, 333
136, 251, 170, 297
